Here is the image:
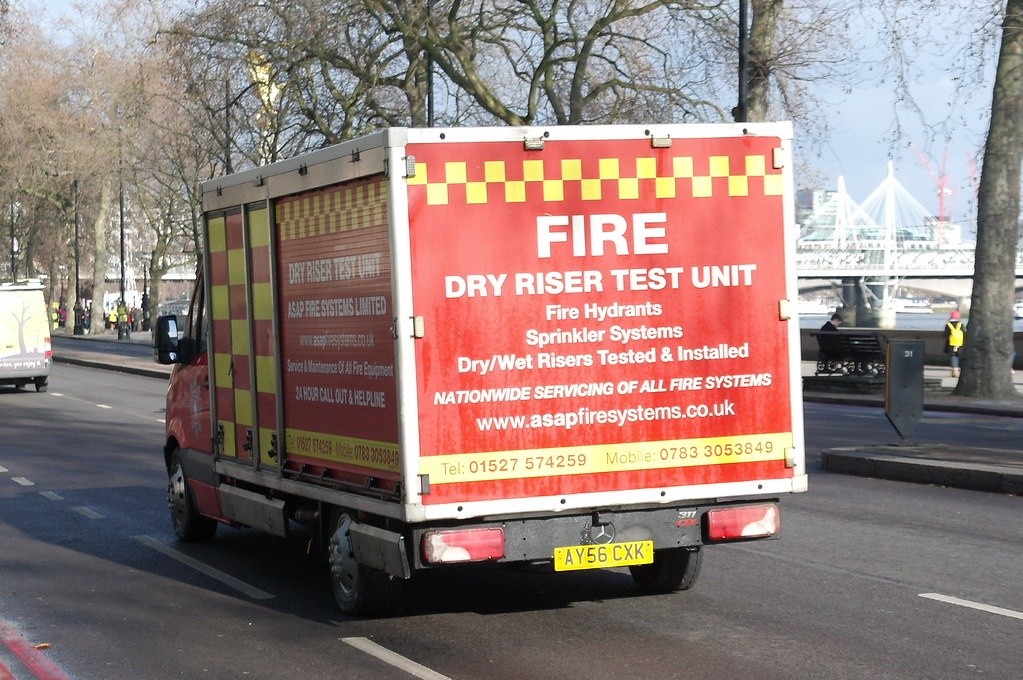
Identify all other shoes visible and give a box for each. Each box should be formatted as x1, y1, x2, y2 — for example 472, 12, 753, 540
952, 368, 960, 378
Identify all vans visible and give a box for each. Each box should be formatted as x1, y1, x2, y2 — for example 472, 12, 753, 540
0, 280, 53, 392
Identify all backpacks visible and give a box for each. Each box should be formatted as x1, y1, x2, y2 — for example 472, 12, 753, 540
948, 322, 963, 347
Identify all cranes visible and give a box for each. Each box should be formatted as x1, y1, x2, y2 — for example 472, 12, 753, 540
906, 140, 950, 222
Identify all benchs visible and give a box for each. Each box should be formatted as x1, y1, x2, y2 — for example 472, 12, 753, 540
811, 332, 888, 376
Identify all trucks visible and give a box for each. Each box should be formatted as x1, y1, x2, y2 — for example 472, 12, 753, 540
151, 121, 810, 617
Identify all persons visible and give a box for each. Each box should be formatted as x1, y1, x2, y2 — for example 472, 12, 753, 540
820, 314, 842, 331
55, 296, 132, 339
943, 311, 965, 378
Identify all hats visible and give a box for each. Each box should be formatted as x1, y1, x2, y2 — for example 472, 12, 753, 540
950, 311, 960, 319
